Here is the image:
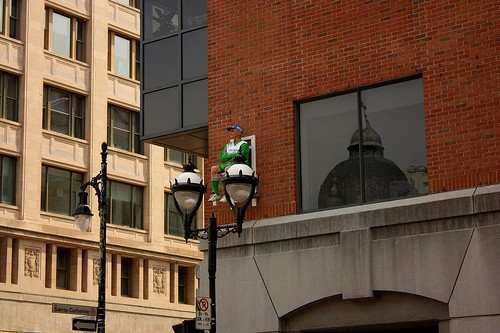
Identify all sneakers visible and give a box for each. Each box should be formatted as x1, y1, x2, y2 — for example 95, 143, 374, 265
208, 193, 221, 202
219, 195, 228, 202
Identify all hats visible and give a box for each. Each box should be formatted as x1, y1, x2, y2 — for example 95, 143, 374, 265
227, 124, 243, 134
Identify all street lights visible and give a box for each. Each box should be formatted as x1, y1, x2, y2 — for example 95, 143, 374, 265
71, 139, 109, 333
169, 145, 261, 333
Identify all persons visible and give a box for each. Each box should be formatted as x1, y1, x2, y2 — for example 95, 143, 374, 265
208, 125, 250, 204
